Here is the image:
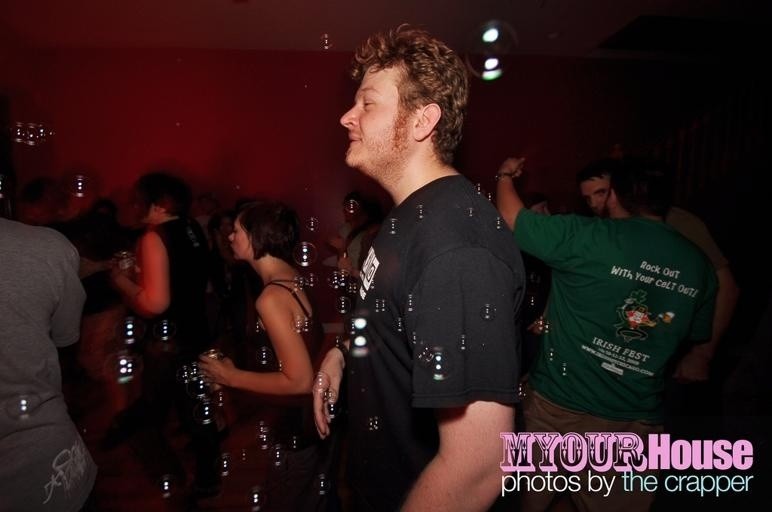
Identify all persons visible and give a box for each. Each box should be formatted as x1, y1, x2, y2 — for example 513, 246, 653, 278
1, 154, 772, 512
305, 22, 526, 512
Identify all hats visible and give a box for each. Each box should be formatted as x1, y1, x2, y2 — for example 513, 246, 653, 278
139, 171, 192, 214
